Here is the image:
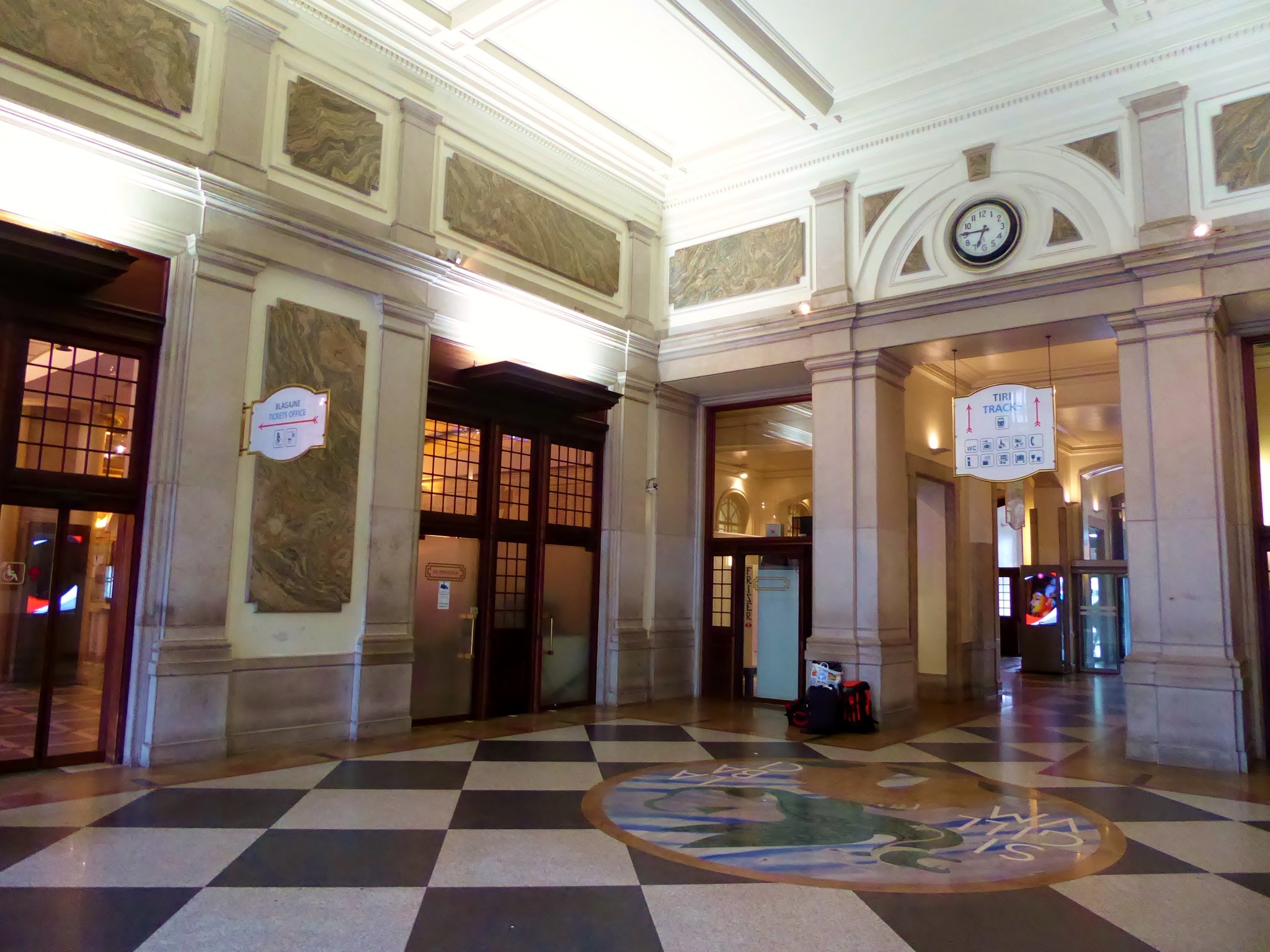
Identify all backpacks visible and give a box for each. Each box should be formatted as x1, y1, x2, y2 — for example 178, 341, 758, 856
837, 679, 880, 735
804, 683, 838, 735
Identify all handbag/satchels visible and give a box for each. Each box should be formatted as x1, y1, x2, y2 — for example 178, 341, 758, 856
809, 660, 843, 689
784, 698, 810, 727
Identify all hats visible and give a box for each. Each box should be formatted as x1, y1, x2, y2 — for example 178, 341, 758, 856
1031, 574, 1056, 602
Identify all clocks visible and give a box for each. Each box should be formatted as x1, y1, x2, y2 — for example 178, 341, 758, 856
943, 191, 1030, 274
